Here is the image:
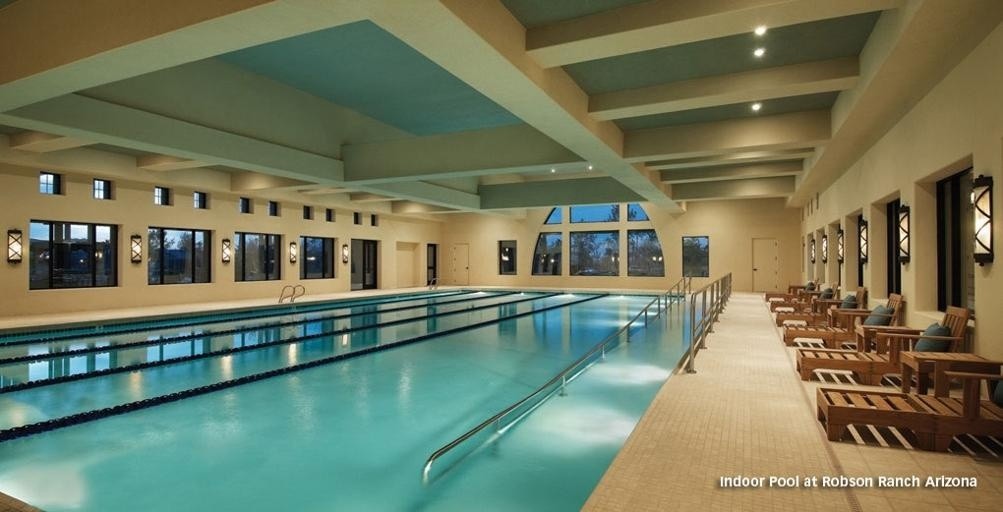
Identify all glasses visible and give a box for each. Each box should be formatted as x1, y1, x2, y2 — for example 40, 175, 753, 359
290, 242, 297, 263
972, 174, 993, 265
898, 204, 909, 265
811, 234, 827, 263
343, 244, 348, 263
859, 219, 868, 263
8, 228, 22, 263
222, 239, 230, 263
837, 229, 843, 263
131, 234, 142, 263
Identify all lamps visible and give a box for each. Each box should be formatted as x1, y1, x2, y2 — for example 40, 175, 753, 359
131, 234, 142, 263
222, 239, 230, 263
859, 219, 868, 263
290, 242, 297, 263
343, 244, 348, 263
972, 174, 993, 265
898, 204, 909, 265
837, 229, 843, 263
8, 228, 22, 263
811, 234, 827, 263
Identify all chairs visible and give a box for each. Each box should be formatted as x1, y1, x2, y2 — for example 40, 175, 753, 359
766, 277, 1002, 451
